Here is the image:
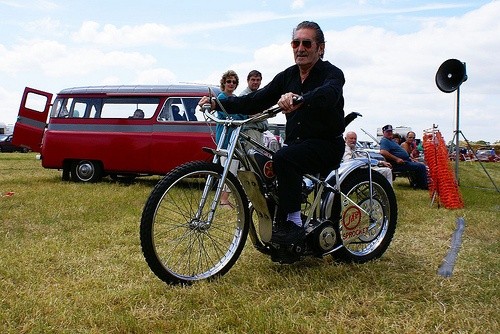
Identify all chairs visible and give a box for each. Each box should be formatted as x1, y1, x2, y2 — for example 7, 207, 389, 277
378, 138, 419, 189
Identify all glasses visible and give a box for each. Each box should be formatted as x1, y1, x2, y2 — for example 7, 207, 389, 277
409, 137, 415, 139
291, 40, 319, 48
226, 80, 237, 84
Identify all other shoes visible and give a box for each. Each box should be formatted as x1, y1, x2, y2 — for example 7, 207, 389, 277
220, 203, 235, 209
271, 220, 304, 240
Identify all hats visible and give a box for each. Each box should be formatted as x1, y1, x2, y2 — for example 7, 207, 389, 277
382, 125, 394, 131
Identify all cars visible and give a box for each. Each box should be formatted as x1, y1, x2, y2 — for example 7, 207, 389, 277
0, 134, 14, 152
451, 146, 500, 162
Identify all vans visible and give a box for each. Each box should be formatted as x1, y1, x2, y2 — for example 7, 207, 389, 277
11, 84, 277, 183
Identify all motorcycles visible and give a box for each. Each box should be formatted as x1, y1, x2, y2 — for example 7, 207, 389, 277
140, 95, 398, 287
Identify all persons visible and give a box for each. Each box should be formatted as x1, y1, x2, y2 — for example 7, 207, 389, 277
215, 70, 268, 210
62, 110, 80, 181
380, 125, 431, 190
128, 109, 144, 120
342, 131, 393, 189
451, 146, 474, 166
171, 104, 197, 122
490, 148, 496, 163
198, 21, 346, 244
